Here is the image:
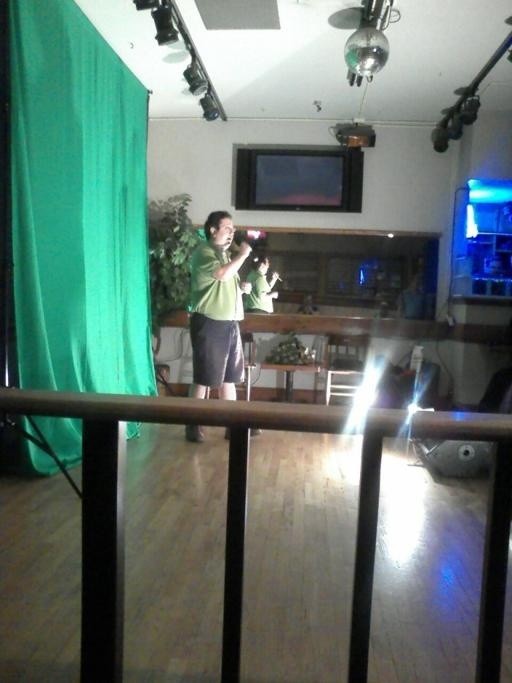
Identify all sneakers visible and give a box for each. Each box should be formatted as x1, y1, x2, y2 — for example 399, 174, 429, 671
225, 428, 262, 435
186, 425, 205, 443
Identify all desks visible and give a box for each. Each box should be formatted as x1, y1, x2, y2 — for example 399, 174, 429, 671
260, 362, 321, 402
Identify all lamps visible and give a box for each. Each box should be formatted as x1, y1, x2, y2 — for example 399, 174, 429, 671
433, 18, 512, 152
131, 0, 231, 119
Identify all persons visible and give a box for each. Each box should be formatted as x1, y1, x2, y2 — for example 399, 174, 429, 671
297, 295, 319, 314
243, 253, 281, 314
184, 209, 264, 444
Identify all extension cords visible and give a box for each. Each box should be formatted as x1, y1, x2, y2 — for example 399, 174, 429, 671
446, 313, 455, 328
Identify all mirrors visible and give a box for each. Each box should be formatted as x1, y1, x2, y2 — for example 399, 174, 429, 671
227, 228, 441, 326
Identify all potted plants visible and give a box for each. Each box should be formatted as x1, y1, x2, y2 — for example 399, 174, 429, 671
148, 193, 202, 324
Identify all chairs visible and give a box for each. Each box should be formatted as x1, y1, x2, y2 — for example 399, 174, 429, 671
206, 332, 254, 404
324, 335, 373, 407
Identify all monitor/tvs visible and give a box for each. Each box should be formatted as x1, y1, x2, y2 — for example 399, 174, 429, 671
233, 148, 364, 214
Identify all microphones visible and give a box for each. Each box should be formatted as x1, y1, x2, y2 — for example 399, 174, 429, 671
268, 267, 282, 283
234, 233, 259, 264
